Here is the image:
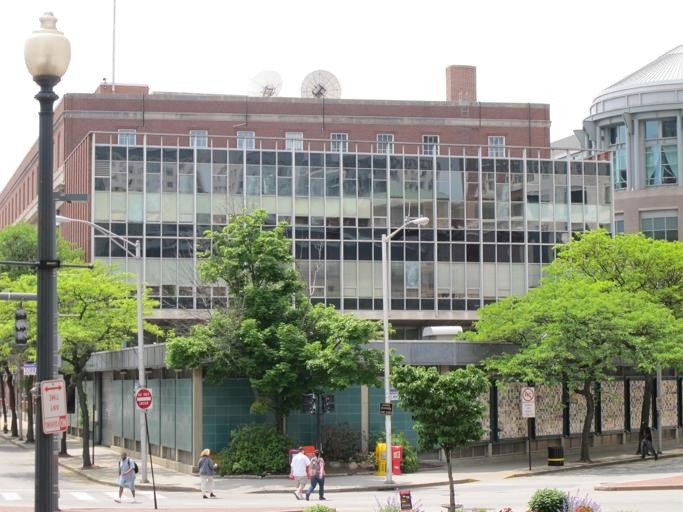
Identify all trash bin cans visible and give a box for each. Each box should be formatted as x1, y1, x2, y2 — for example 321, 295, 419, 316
548, 447, 564, 466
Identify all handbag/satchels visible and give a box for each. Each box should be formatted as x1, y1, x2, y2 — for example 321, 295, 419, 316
191, 465, 200, 474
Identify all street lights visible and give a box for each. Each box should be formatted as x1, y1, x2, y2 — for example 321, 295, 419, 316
378, 216, 433, 485
16, 11, 73, 511
50, 214, 151, 483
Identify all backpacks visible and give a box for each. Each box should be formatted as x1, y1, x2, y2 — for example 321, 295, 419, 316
118, 458, 138, 473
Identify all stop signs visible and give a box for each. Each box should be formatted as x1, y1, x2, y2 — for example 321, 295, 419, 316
134, 387, 154, 411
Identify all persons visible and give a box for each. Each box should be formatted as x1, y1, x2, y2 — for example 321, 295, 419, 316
111, 449, 137, 504
304, 449, 326, 501
639, 419, 659, 461
289, 446, 311, 500
197, 447, 217, 499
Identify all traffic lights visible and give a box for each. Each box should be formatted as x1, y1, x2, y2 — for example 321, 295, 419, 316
302, 392, 319, 415
13, 306, 27, 347
318, 392, 337, 415
